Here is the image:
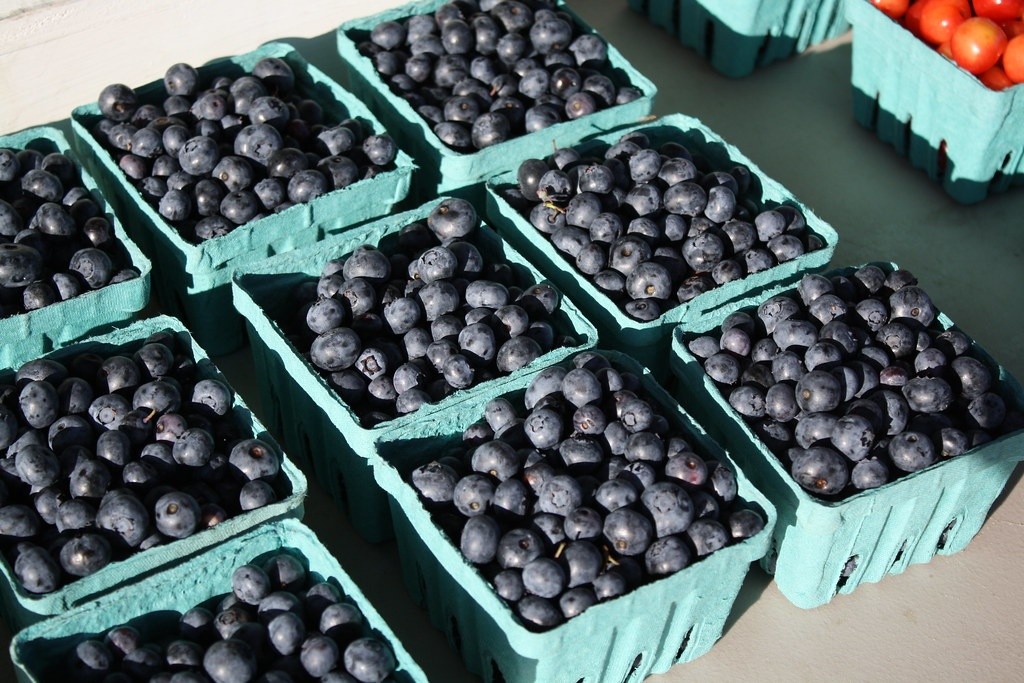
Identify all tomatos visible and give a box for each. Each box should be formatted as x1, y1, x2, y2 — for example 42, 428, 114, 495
870, 0, 1024, 92
